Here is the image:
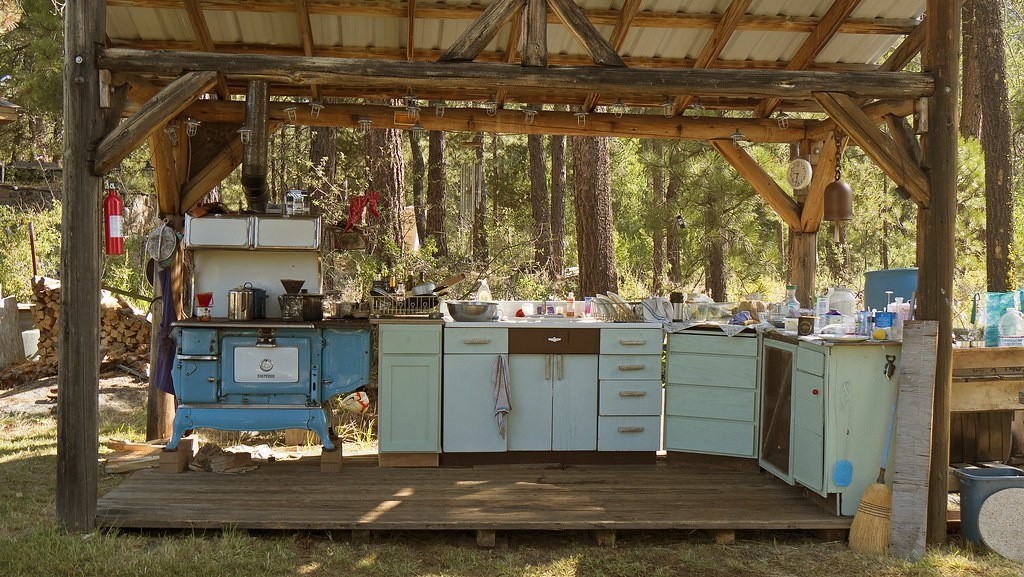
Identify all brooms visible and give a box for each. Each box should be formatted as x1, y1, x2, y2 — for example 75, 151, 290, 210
848, 364, 910, 555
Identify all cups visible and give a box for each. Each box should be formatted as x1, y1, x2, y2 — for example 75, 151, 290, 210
195, 305, 213, 322
584, 296, 594, 317
282, 294, 304, 322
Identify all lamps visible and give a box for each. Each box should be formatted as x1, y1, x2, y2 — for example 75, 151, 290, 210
165, 109, 789, 146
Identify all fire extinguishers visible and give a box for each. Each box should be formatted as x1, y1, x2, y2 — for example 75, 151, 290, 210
104, 182, 124, 255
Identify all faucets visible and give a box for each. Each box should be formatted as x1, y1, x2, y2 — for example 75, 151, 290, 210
541, 298, 546, 315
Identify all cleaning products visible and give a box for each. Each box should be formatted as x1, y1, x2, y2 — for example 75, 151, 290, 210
477, 279, 492, 302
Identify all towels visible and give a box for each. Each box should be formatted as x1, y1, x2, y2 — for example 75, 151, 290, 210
641, 298, 673, 324
490, 354, 513, 441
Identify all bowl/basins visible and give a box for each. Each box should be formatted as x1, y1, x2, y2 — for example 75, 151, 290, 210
340, 391, 370, 415
757, 312, 820, 336
683, 300, 708, 323
445, 301, 499, 322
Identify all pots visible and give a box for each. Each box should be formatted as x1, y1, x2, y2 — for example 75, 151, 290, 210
281, 289, 324, 323
397, 271, 449, 310
228, 282, 267, 323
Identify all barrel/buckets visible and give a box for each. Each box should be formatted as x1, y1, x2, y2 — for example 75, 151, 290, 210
862, 267, 918, 313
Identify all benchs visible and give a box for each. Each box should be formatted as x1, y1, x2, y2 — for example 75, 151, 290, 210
8, 159, 62, 180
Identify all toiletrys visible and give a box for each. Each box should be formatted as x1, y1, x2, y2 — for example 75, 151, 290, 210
566, 292, 575, 318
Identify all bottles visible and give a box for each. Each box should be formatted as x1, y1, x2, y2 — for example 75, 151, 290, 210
476, 280, 492, 301
998, 308, 1024, 348
284, 190, 304, 215
783, 284, 801, 319
566, 292, 575, 318
815, 285, 912, 342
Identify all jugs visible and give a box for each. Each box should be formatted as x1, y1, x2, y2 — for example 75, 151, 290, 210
670, 292, 685, 322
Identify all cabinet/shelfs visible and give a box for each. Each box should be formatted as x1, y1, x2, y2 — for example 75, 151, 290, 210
378, 324, 896, 517
164, 212, 374, 451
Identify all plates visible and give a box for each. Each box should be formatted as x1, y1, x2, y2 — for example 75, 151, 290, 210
591, 291, 631, 320
817, 334, 870, 343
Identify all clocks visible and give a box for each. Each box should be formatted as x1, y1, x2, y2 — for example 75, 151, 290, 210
788, 159, 812, 190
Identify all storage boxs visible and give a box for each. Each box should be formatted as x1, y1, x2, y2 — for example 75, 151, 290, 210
997, 338, 1024, 347
875, 312, 898, 337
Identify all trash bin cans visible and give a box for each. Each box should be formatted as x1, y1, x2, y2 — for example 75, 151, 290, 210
953, 467, 1024, 548
